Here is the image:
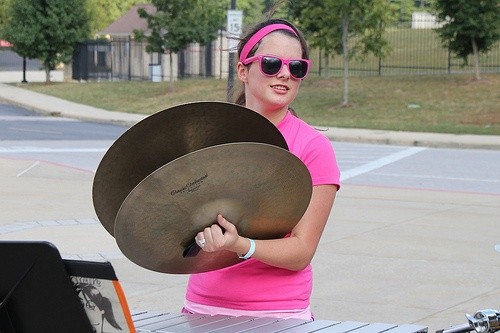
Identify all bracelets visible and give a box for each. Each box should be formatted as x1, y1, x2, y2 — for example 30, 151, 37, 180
237, 237, 256, 260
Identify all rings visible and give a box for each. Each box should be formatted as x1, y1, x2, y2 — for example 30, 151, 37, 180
199, 239, 205, 245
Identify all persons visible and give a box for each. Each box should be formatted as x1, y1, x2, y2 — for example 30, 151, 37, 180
182, 18, 341, 322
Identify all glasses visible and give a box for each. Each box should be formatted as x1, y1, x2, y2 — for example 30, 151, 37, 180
243, 55, 310, 79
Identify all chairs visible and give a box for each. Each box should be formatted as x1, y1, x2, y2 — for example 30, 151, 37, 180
0, 240, 136, 333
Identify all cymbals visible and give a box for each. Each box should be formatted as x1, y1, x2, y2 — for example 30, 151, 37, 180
90, 101, 290, 240
112, 140, 314, 275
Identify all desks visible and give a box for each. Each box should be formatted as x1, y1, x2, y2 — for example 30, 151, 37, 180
130, 309, 428, 333
